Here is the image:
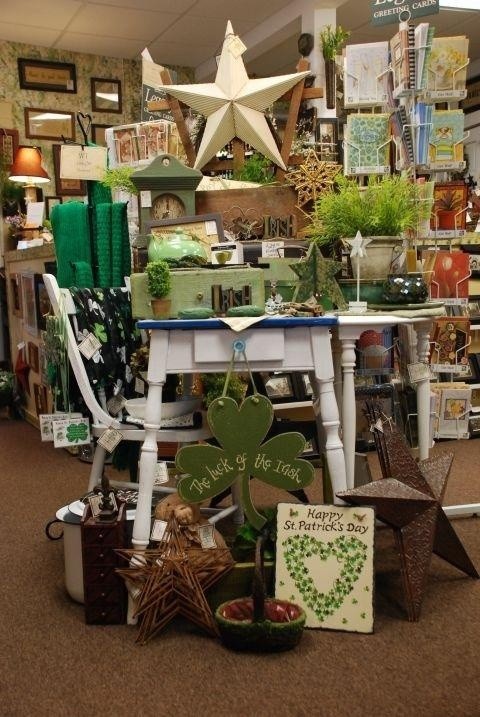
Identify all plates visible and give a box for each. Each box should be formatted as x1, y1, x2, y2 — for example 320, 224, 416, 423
68, 497, 86, 517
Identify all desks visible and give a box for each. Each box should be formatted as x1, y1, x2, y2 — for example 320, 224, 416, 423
126, 313, 432, 628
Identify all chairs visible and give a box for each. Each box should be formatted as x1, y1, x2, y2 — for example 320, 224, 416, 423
41, 269, 243, 515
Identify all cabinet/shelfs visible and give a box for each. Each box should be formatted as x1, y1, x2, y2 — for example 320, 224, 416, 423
326, 23, 475, 447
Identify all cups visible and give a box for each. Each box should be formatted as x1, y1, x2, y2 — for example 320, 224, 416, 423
55, 504, 85, 604
215, 252, 228, 264
222, 250, 233, 261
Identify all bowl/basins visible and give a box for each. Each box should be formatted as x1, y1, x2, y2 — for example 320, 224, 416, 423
124, 395, 198, 421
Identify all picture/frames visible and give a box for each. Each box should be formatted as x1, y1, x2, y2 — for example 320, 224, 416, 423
22, 104, 78, 144
89, 122, 119, 147
1, 127, 21, 172
15, 55, 79, 96
87, 75, 125, 115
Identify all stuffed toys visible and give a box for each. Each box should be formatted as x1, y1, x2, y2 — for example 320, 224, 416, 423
154, 491, 236, 570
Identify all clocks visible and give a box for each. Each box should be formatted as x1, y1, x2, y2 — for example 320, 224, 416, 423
126, 153, 205, 249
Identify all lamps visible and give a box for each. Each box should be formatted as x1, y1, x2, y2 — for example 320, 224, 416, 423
7, 146, 52, 214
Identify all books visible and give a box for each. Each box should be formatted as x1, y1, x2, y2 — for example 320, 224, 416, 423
104, 118, 178, 167
430, 379, 475, 442
338, 19, 471, 235
429, 249, 470, 299
428, 315, 472, 374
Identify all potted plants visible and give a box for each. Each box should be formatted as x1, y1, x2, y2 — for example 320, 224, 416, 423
304, 167, 434, 282
